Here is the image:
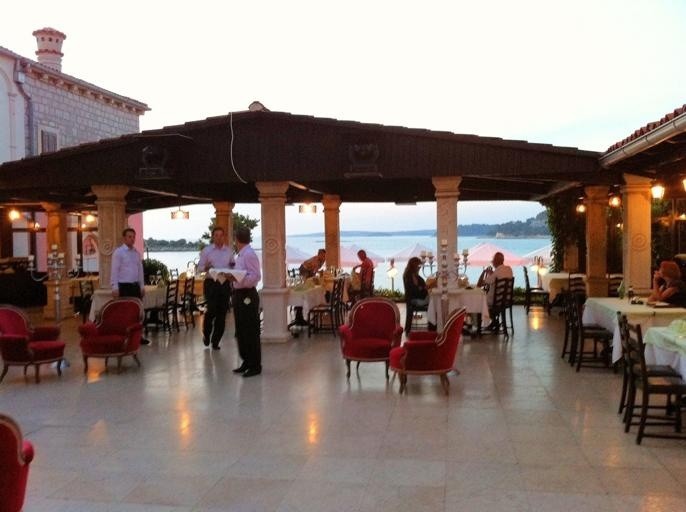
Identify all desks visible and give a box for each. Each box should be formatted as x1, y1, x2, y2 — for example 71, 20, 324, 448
542, 274, 624, 316
643, 327, 686, 419
89, 285, 177, 327
258, 285, 323, 338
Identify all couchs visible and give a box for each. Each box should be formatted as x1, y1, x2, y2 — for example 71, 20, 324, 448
338, 296, 403, 380
0, 304, 65, 387
0, 414, 35, 512
78, 297, 145, 374
391, 306, 472, 396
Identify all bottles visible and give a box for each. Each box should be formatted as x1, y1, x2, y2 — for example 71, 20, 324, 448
628, 281, 634, 302
619, 282, 625, 298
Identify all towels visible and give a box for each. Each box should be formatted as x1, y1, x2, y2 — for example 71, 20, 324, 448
208, 267, 248, 285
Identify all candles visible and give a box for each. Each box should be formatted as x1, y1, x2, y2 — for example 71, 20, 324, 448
453, 254, 459, 259
51, 244, 58, 251
463, 249, 467, 254
75, 254, 80, 260
58, 252, 64, 259
28, 255, 34, 262
428, 252, 433, 258
48, 254, 52, 260
421, 251, 426, 257
441, 239, 446, 246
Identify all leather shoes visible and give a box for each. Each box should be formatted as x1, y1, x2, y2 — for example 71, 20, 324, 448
141, 336, 150, 345
212, 343, 221, 351
203, 336, 210, 345
233, 365, 248, 372
242, 366, 262, 377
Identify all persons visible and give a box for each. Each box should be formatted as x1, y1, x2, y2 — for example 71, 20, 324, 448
299, 249, 326, 283
468, 252, 514, 336
198, 227, 235, 351
110, 228, 152, 347
404, 257, 437, 308
352, 250, 374, 298
223, 227, 262, 378
647, 261, 685, 306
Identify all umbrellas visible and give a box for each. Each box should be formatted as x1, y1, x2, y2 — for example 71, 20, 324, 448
516, 240, 555, 267
255, 244, 311, 265
388, 242, 437, 263
459, 243, 529, 273
459, 242, 486, 259
350, 243, 385, 264
339, 244, 379, 269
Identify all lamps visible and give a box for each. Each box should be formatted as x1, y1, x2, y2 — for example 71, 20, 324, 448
538, 257, 548, 283
298, 203, 317, 213
576, 197, 585, 213
529, 255, 540, 283
651, 176, 665, 199
171, 206, 189, 219
607, 184, 621, 206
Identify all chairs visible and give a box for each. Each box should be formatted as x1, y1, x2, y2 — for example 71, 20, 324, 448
287, 268, 300, 311
145, 280, 180, 336
319, 278, 345, 331
625, 323, 686, 445
562, 289, 606, 363
79, 279, 94, 323
170, 268, 178, 280
403, 285, 434, 336
524, 266, 550, 316
172, 277, 195, 330
570, 297, 613, 372
351, 264, 363, 307
308, 278, 340, 337
569, 271, 586, 292
524, 265, 545, 311
605, 271, 623, 296
616, 312, 682, 421
487, 277, 514, 338
